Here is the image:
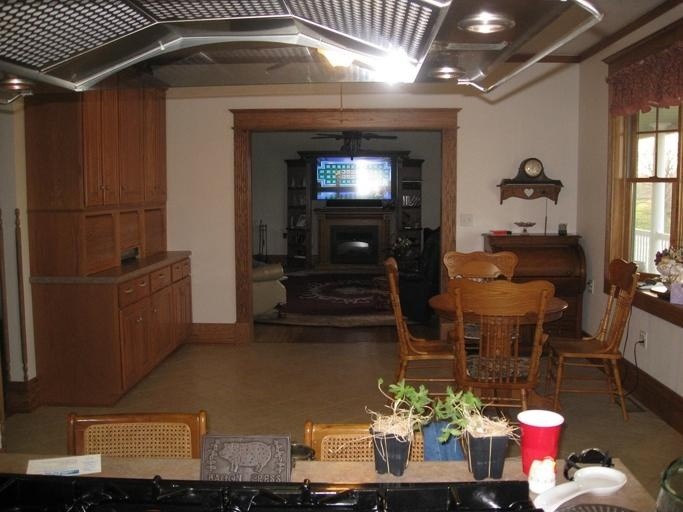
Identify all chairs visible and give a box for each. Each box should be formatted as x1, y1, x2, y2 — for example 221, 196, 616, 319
66, 409, 207, 458
547, 258, 638, 421
303, 420, 425, 461
385, 257, 455, 410
448, 279, 555, 425
443, 251, 519, 282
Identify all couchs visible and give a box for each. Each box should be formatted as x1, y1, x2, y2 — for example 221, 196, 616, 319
252, 263, 287, 319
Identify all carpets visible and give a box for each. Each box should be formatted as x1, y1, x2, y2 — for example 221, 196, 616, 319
253, 270, 395, 328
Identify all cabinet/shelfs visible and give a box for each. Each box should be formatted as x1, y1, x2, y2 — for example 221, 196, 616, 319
482, 233, 587, 339
283, 159, 311, 263
399, 158, 425, 252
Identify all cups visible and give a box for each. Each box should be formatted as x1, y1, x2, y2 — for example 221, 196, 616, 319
517, 408, 566, 477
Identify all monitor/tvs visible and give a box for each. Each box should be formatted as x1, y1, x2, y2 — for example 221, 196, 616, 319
313, 154, 396, 208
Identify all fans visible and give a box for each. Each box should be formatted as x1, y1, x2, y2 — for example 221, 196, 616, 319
311, 131, 397, 160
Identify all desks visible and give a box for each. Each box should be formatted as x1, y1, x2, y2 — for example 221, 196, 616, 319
0, 452, 659, 512
429, 293, 568, 412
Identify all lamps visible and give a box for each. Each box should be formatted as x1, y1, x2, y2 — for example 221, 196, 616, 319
2, 79, 35, 90
457, 12, 516, 34
425, 67, 465, 80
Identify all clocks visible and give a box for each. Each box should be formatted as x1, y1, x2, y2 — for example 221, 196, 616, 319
496, 158, 564, 205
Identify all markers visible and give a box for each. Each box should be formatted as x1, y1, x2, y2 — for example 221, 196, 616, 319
492, 230, 512, 235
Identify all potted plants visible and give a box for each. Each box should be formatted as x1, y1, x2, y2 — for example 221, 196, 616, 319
362, 378, 434, 476
434, 386, 519, 479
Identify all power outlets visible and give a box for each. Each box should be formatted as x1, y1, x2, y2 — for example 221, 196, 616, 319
639, 330, 648, 348
